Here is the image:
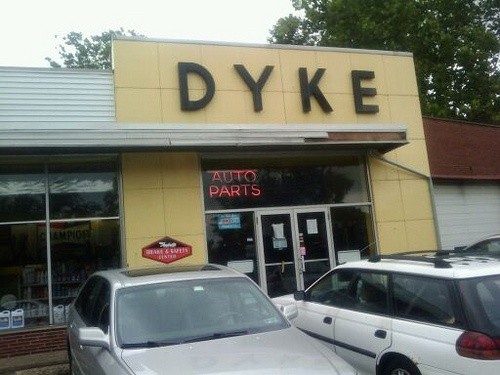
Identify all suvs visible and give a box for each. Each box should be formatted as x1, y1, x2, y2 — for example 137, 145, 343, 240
272, 249, 500, 375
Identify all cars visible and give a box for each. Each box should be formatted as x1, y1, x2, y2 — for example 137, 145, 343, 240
65, 262, 362, 375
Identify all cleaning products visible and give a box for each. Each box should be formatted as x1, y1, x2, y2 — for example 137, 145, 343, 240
52, 305, 70, 325
0, 308, 25, 330
